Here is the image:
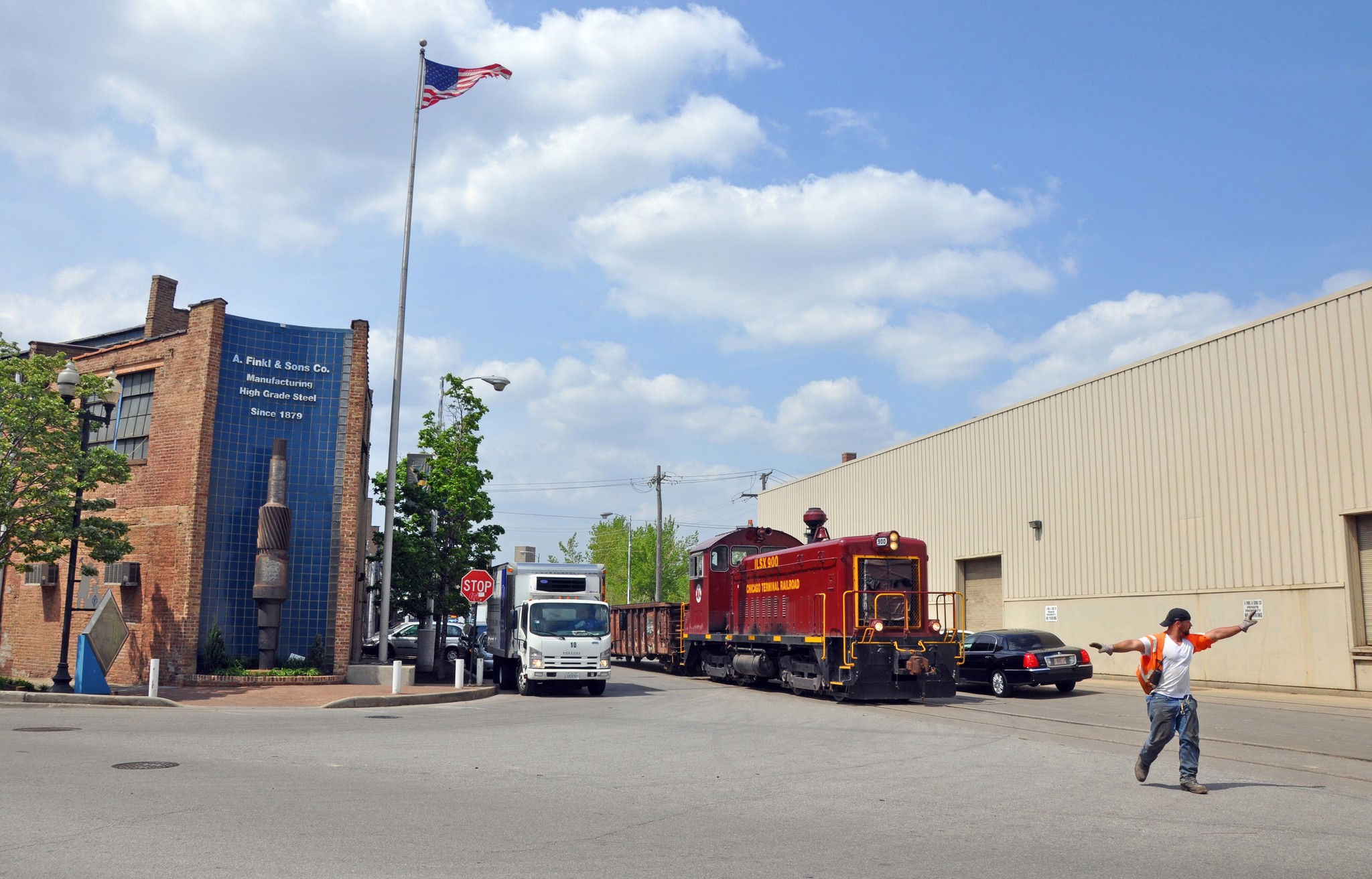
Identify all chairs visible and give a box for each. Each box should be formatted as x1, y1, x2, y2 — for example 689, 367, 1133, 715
533, 609, 546, 630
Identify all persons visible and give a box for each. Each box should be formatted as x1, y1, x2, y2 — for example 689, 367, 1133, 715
1089, 608, 1258, 794
566, 608, 607, 631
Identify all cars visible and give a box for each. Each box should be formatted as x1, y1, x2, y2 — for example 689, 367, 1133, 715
959, 627, 1093, 698
361, 615, 464, 663
938, 630, 977, 649
472, 632, 493, 676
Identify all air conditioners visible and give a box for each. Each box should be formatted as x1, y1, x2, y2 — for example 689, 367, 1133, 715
23, 563, 59, 587
103, 561, 140, 586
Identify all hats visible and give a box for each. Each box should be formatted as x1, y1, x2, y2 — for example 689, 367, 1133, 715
1160, 608, 1191, 627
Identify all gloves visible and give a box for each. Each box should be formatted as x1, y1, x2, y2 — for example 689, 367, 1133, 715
1238, 610, 1258, 633
1089, 642, 1114, 656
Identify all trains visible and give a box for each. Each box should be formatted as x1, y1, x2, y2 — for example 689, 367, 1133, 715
602, 507, 962, 703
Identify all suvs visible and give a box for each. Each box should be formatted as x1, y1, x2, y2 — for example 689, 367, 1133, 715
456, 622, 487, 671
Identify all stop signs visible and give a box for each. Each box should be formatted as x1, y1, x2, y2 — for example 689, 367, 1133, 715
461, 569, 495, 603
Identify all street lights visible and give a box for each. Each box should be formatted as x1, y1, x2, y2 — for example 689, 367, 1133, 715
424, 375, 511, 630
520, 550, 540, 563
46, 357, 124, 694
600, 512, 632, 604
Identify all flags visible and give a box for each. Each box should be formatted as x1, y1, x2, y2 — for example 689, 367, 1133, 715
420, 57, 513, 109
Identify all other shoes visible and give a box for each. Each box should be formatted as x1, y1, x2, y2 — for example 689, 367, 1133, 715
1135, 755, 1149, 782
1181, 779, 1207, 793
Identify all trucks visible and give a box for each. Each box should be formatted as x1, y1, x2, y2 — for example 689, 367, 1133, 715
485, 561, 628, 697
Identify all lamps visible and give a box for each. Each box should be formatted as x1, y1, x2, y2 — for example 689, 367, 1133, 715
1028, 520, 1041, 529
